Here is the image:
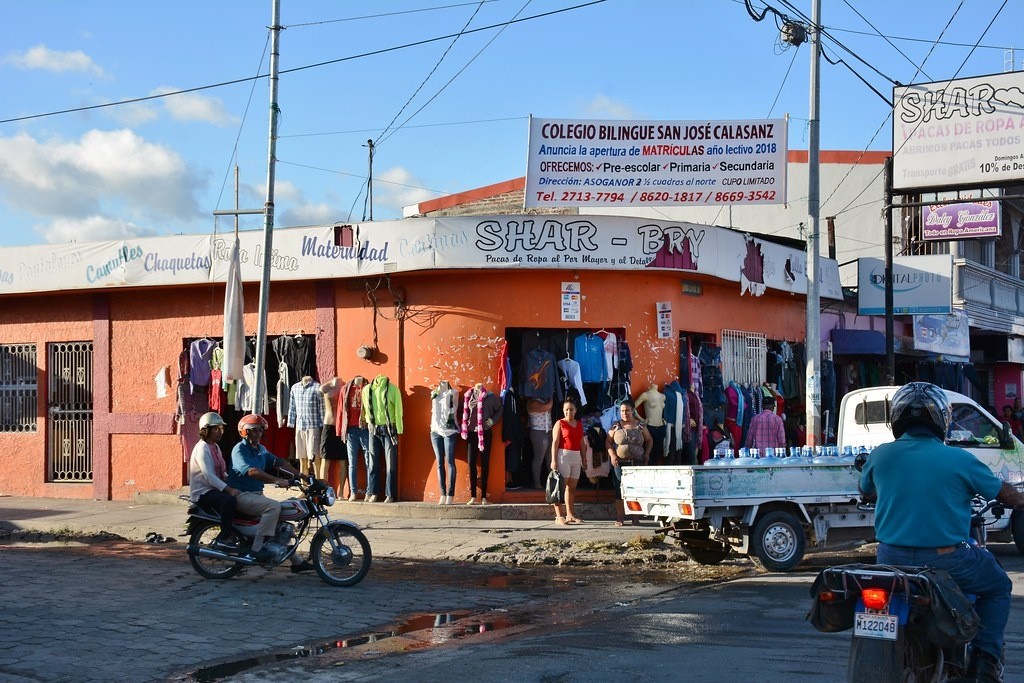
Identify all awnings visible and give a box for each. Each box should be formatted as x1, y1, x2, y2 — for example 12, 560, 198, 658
831, 329, 937, 356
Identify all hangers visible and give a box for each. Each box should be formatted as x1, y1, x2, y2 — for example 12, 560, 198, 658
282, 329, 286, 338
701, 337, 721, 346
201, 332, 208, 341
213, 363, 219, 371
535, 327, 610, 362
250, 332, 257, 341
280, 356, 285, 365
189, 405, 198, 414
295, 329, 303, 339
251, 357, 255, 366
678, 337, 686, 341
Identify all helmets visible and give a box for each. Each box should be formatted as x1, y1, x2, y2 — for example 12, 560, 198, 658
238, 414, 268, 437
889, 381, 950, 441
199, 412, 226, 430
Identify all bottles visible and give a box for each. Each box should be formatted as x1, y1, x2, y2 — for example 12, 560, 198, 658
704, 446, 877, 465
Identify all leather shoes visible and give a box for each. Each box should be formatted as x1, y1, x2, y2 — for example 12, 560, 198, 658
967, 657, 1003, 683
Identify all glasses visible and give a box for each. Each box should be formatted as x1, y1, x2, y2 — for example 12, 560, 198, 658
250, 428, 264, 432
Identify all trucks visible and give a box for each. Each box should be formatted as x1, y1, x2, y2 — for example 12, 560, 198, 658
619, 380, 1024, 574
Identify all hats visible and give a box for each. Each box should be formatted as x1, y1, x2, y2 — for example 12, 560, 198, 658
762, 396, 775, 404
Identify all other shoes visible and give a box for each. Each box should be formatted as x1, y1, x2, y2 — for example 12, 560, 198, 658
217, 539, 237, 549
249, 543, 282, 557
290, 560, 316, 572
506, 481, 522, 489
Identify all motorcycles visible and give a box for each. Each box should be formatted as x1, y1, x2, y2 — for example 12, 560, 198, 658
820, 452, 1024, 683
175, 453, 373, 588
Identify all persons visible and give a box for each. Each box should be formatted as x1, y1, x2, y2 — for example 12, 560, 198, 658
430, 380, 460, 505
503, 391, 523, 490
190, 412, 317, 573
583, 427, 611, 485
527, 394, 553, 490
287, 376, 403, 503
985, 397, 1024, 445
858, 382, 1024, 683
460, 383, 503, 505
550, 396, 588, 524
605, 400, 653, 527
703, 381, 786, 459
791, 425, 806, 447
633, 381, 703, 466
745, 396, 787, 458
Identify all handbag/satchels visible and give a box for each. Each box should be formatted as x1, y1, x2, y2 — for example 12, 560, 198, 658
920, 568, 982, 646
805, 563, 862, 633
545, 470, 565, 504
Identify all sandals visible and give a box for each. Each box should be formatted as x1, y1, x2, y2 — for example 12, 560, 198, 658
566, 515, 584, 524
555, 517, 568, 526
